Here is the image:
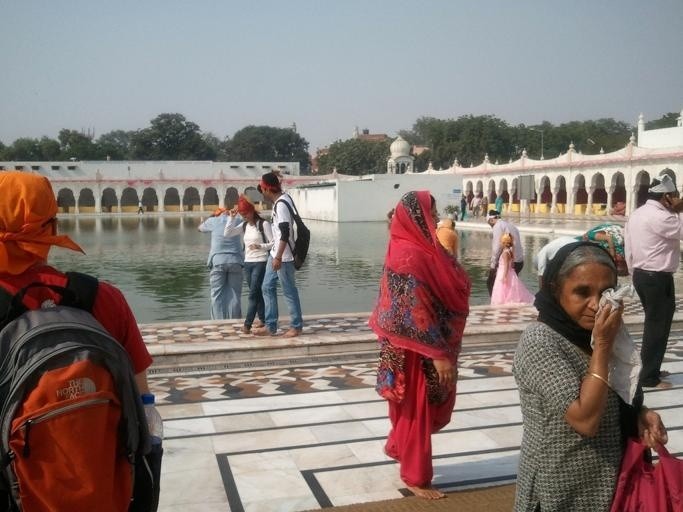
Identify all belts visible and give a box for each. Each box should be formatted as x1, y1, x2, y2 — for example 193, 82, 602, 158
633, 266, 675, 278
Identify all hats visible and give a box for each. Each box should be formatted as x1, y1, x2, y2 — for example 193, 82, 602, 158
259, 173, 281, 192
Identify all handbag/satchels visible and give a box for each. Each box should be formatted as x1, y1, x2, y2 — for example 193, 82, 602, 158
609, 434, 683, 512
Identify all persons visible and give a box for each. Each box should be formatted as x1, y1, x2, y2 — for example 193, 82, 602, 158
624, 173, 683, 389
368, 192, 472, 500
460, 194, 504, 222
0, 172, 153, 512
575, 223, 627, 276
251, 173, 303, 338
532, 236, 580, 289
490, 232, 535, 305
435, 219, 459, 260
511, 240, 668, 512
197, 207, 243, 320
223, 194, 274, 333
136, 201, 144, 214
486, 209, 524, 297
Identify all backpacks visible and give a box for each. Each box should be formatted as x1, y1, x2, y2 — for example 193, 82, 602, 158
0, 273, 165, 512
274, 194, 310, 270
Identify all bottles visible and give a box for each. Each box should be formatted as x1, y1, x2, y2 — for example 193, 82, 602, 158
138, 392, 163, 461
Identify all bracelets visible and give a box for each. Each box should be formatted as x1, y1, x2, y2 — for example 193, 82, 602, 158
274, 257, 282, 261
584, 371, 612, 389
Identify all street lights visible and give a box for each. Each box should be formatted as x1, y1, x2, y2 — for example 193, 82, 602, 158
530, 128, 544, 160
